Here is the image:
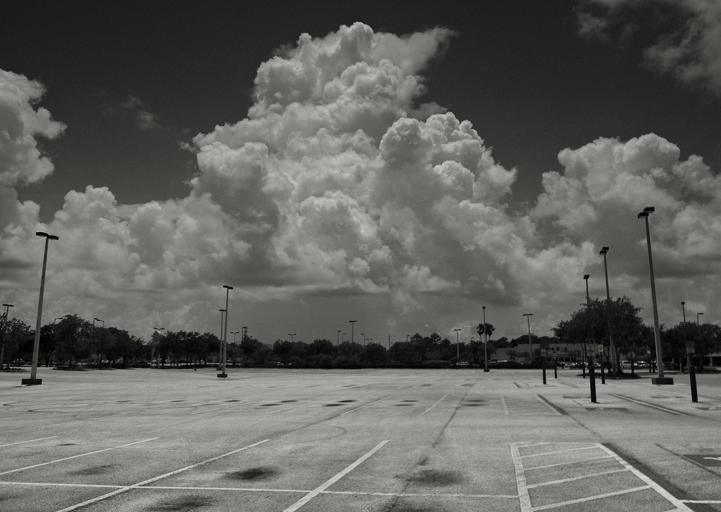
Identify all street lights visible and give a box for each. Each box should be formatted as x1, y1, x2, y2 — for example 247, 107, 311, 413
638, 206, 674, 386
523, 313, 533, 361
216, 308, 227, 371
482, 306, 490, 373
697, 312, 704, 327
583, 274, 592, 343
216, 285, 233, 379
21, 231, 59, 385
2, 303, 14, 329
599, 247, 618, 378
349, 320, 357, 344
681, 301, 686, 322
454, 328, 462, 363
